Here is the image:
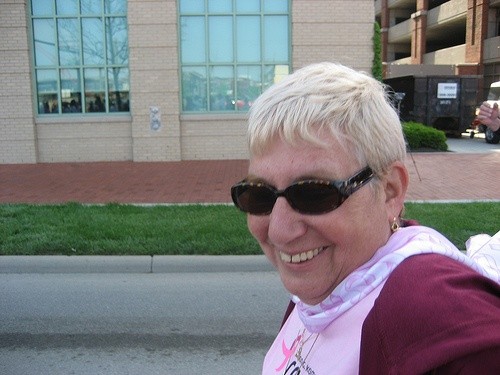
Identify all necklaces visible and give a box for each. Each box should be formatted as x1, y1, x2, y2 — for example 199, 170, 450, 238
284, 328, 320, 375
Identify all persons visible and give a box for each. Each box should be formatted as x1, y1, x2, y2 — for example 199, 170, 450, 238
231, 61, 500, 375
477, 100, 500, 145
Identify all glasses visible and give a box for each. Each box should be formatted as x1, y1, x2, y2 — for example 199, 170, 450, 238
230, 165, 376, 217
